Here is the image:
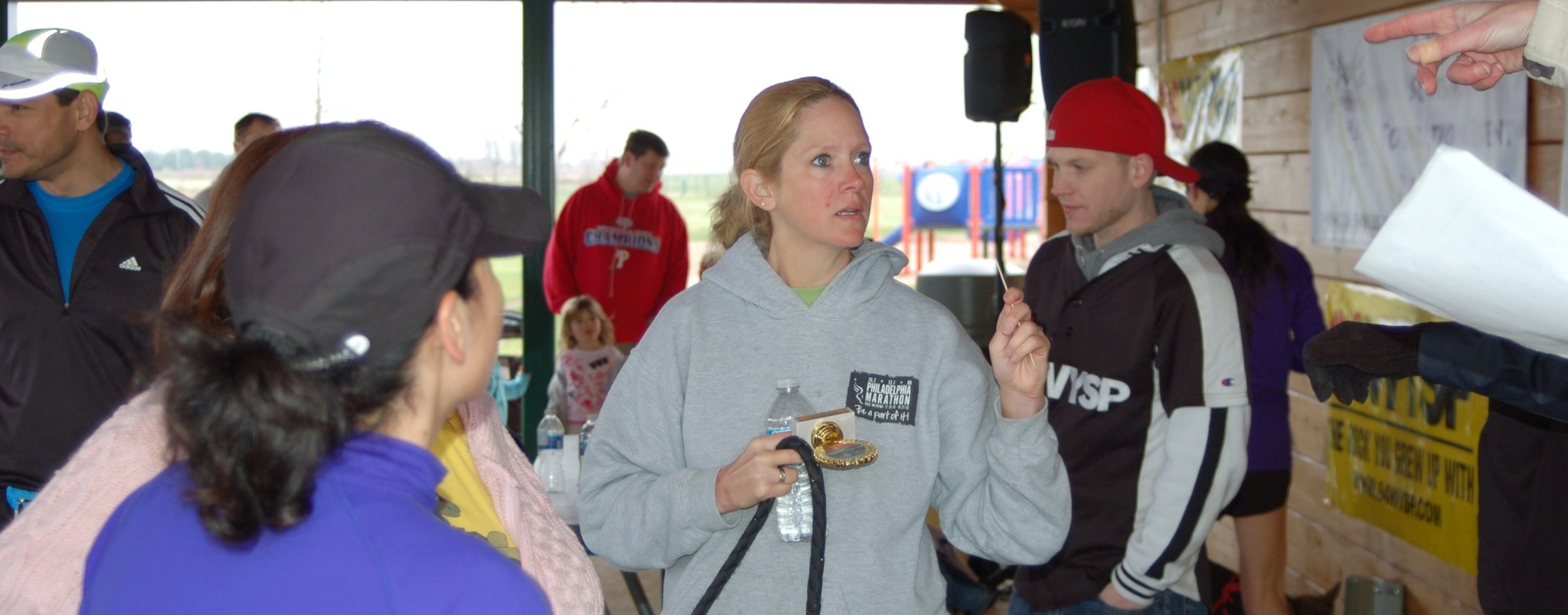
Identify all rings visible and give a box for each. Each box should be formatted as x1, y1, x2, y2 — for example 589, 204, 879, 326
777, 467, 785, 483
1462, 54, 1475, 63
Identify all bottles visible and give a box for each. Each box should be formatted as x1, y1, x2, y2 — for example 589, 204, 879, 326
577, 414, 598, 492
765, 376, 829, 543
537, 409, 566, 492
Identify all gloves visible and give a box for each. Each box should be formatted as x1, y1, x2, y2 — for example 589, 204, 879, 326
1302, 320, 1427, 406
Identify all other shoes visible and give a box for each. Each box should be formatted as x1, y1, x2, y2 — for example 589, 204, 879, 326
935, 549, 997, 615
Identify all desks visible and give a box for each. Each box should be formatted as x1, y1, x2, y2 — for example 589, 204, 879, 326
533, 434, 655, 615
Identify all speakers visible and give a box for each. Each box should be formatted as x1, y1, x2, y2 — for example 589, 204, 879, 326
1037, 0, 1140, 115
961, 9, 1032, 123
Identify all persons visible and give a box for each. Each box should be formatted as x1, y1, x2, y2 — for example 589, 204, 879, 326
1013, 79, 1250, 615
0, 114, 1328, 615
1303, 0, 1568, 615
0, 29, 216, 534
574, 76, 1073, 615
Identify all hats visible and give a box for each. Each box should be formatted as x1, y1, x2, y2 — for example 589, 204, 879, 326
222, 119, 554, 379
1045, 76, 1201, 183
0, 28, 110, 106
